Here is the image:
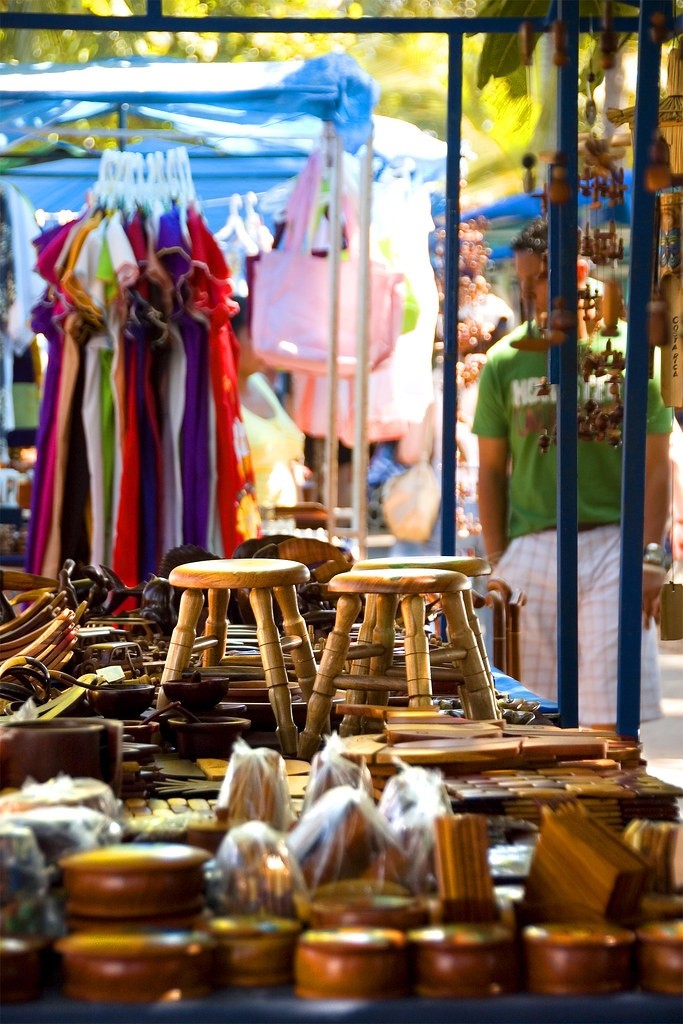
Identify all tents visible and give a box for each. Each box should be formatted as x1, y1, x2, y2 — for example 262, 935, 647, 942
1, 51, 668, 430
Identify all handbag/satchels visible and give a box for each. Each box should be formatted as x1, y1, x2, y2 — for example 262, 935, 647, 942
249, 149, 406, 381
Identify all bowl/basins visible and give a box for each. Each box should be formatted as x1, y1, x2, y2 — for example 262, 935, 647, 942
1, 718, 107, 792
161, 678, 229, 716
88, 684, 155, 719
168, 717, 252, 755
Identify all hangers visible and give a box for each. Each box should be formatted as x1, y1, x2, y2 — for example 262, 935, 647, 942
243, 188, 275, 252
212, 193, 259, 257
89, 146, 200, 220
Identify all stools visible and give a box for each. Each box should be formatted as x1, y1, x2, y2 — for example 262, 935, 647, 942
295, 567, 499, 759
338, 557, 502, 738
156, 557, 332, 758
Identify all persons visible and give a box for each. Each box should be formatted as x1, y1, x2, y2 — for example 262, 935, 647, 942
0, 429, 41, 527
363, 220, 683, 738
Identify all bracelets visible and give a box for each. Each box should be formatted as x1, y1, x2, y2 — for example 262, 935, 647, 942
643, 543, 665, 565
